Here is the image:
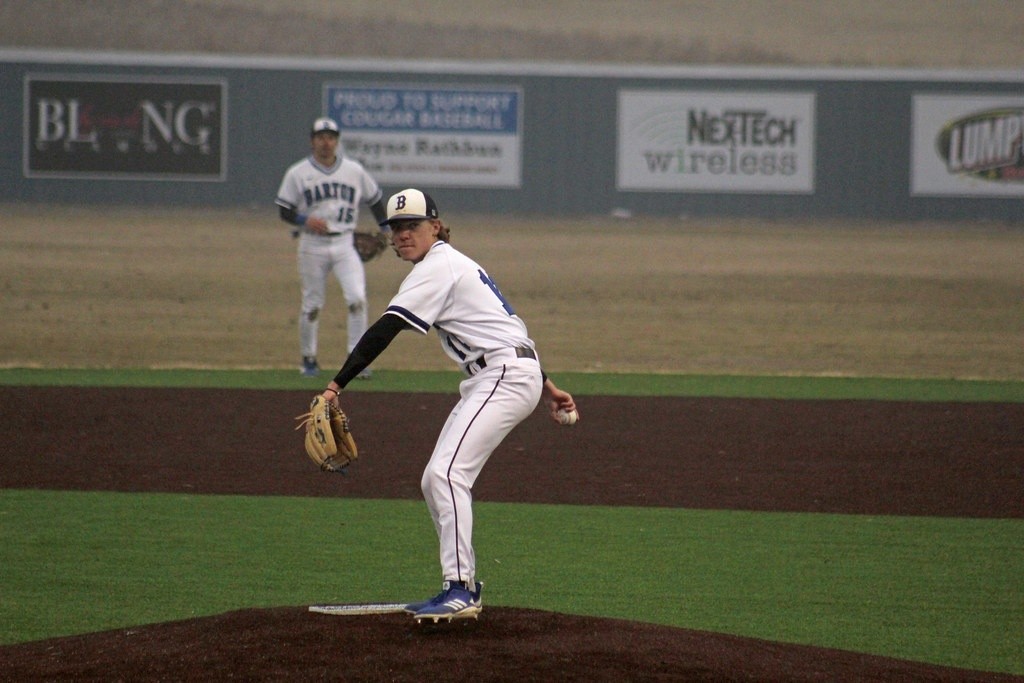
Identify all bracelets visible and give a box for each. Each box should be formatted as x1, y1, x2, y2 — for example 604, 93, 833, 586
326, 388, 342, 398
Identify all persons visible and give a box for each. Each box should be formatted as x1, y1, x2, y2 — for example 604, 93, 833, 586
275, 116, 394, 383
306, 188, 581, 624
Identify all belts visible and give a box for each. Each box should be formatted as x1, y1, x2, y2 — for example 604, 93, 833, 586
466, 348, 537, 375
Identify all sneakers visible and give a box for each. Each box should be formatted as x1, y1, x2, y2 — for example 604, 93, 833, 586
356, 368, 372, 379
402, 581, 483, 614
413, 586, 480, 624
300, 357, 320, 377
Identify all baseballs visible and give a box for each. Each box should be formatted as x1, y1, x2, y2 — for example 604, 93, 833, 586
555, 407, 577, 426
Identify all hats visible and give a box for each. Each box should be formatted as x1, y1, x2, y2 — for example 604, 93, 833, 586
378, 188, 440, 227
311, 117, 340, 138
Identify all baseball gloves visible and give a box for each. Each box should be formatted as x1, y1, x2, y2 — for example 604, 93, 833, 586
294, 394, 359, 478
353, 232, 388, 263
